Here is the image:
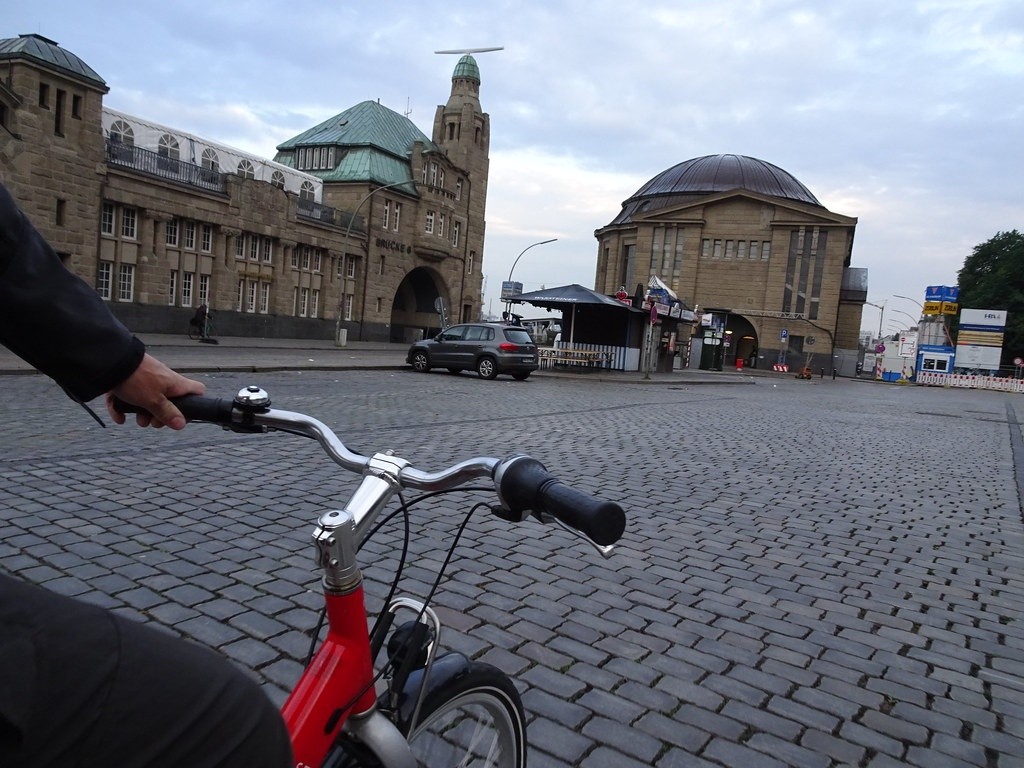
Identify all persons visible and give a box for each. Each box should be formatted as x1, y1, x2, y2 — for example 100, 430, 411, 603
1, 179, 304, 768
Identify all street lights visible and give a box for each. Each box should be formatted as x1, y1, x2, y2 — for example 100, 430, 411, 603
505, 238, 558, 313
334, 178, 420, 346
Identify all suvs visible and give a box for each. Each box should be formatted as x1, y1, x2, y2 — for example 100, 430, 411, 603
406, 322, 539, 380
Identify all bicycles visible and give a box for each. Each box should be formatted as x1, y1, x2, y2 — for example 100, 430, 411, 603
115, 383, 629, 767
189, 317, 218, 340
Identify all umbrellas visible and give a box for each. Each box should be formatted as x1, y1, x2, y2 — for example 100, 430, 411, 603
499, 283, 643, 350
